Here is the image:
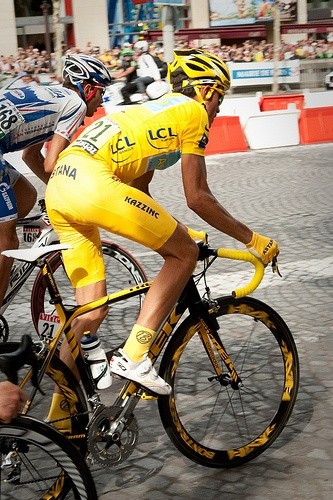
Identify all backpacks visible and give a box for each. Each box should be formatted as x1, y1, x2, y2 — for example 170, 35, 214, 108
144, 53, 168, 80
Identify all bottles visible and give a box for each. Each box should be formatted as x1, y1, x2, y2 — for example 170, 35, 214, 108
79, 332, 113, 390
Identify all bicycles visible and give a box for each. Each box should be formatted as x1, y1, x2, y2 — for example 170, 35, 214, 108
0, 232, 300, 500
0, 198, 150, 369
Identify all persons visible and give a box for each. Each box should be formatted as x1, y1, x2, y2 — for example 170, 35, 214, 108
175, 37, 333, 62
134, 40, 162, 103
0, 54, 110, 300
0, 380, 27, 423
114, 48, 138, 105
45, 48, 279, 436
0, 41, 164, 75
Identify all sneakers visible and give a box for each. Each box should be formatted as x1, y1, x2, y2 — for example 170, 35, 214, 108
108, 348, 172, 396
44, 417, 119, 434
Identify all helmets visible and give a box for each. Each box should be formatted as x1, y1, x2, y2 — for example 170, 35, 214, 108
121, 49, 133, 55
134, 41, 148, 52
168, 48, 231, 90
62, 54, 112, 86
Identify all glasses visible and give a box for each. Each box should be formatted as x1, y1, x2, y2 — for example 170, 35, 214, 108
91, 86, 106, 96
218, 94, 223, 106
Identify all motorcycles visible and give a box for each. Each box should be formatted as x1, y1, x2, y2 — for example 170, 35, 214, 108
101, 76, 171, 107
